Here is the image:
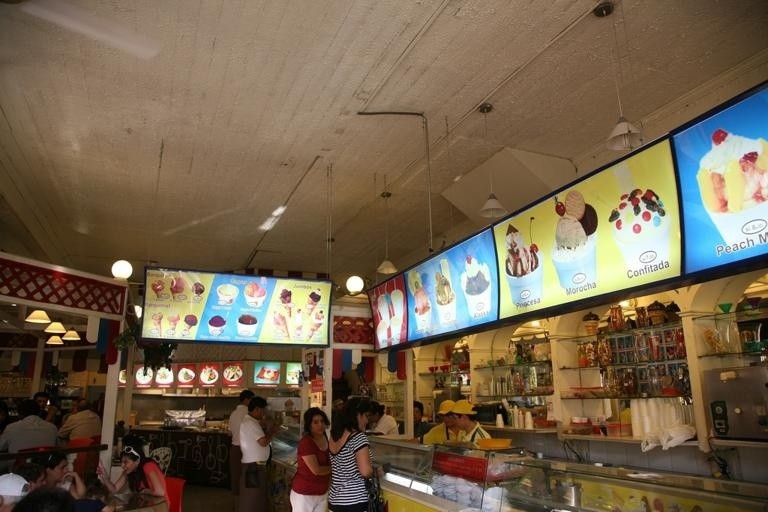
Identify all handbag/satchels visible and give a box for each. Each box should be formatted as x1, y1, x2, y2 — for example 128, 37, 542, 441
364, 474, 384, 512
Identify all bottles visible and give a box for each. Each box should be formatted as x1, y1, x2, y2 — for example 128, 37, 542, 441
551, 478, 583, 507
511, 406, 524, 429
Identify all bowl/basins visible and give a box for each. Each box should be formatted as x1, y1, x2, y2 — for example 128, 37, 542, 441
412, 266, 492, 332
207, 283, 267, 337
506, 214, 671, 307
477, 438, 511, 451
428, 367, 438, 372
206, 419, 222, 428
440, 365, 450, 372
164, 408, 207, 419
701, 200, 768, 247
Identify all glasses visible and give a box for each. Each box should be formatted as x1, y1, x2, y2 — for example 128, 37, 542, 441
119, 446, 140, 459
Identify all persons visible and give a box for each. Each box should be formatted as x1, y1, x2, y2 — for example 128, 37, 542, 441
324, 396, 376, 511
445, 399, 492, 447
237, 394, 280, 511
331, 398, 346, 413
1, 393, 170, 512
364, 401, 402, 465
286, 406, 332, 511
403, 396, 457, 447
412, 399, 433, 438
228, 390, 256, 498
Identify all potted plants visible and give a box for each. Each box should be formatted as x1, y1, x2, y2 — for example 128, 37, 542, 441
112, 316, 179, 377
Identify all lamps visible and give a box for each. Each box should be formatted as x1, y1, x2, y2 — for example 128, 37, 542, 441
477, 103, 508, 219
593, 3, 646, 152
376, 192, 399, 275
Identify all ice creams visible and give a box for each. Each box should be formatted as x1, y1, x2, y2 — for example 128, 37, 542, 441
550, 190, 598, 293
151, 279, 165, 298
308, 288, 321, 314
695, 128, 768, 252
280, 289, 291, 317
306, 310, 324, 339
460, 255, 490, 317
152, 313, 162, 336
192, 282, 203, 301
182, 315, 197, 336
504, 223, 544, 307
168, 313, 179, 336
434, 273, 456, 326
608, 188, 671, 277
414, 288, 432, 331
169, 278, 184, 302
273, 312, 289, 339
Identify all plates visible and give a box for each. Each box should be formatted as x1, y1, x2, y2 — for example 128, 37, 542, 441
184, 426, 197, 428
119, 366, 243, 383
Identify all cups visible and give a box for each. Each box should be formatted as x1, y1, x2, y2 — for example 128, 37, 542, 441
496, 414, 503, 428
571, 415, 606, 425
630, 398, 686, 441
525, 411, 534, 428
717, 302, 732, 312
376, 289, 405, 349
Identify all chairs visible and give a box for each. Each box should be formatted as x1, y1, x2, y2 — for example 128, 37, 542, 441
12, 433, 187, 512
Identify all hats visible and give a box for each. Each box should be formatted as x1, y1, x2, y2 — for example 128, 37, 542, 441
0, 472, 30, 497
436, 399, 455, 415
451, 400, 477, 415
